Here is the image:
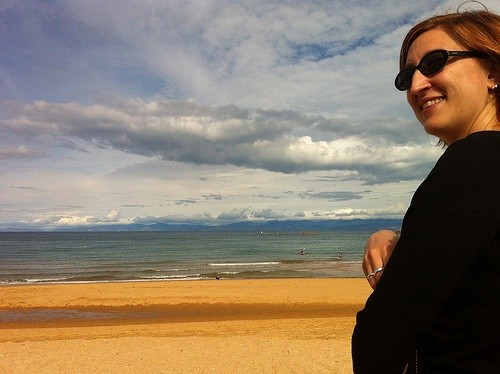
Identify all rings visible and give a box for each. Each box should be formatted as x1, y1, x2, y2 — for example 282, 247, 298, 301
374, 268, 383, 277
367, 273, 375, 279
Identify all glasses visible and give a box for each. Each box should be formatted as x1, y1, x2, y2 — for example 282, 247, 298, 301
395, 49, 484, 91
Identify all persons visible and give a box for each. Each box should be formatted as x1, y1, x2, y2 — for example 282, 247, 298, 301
351, 1, 500, 374
300, 248, 305, 254
